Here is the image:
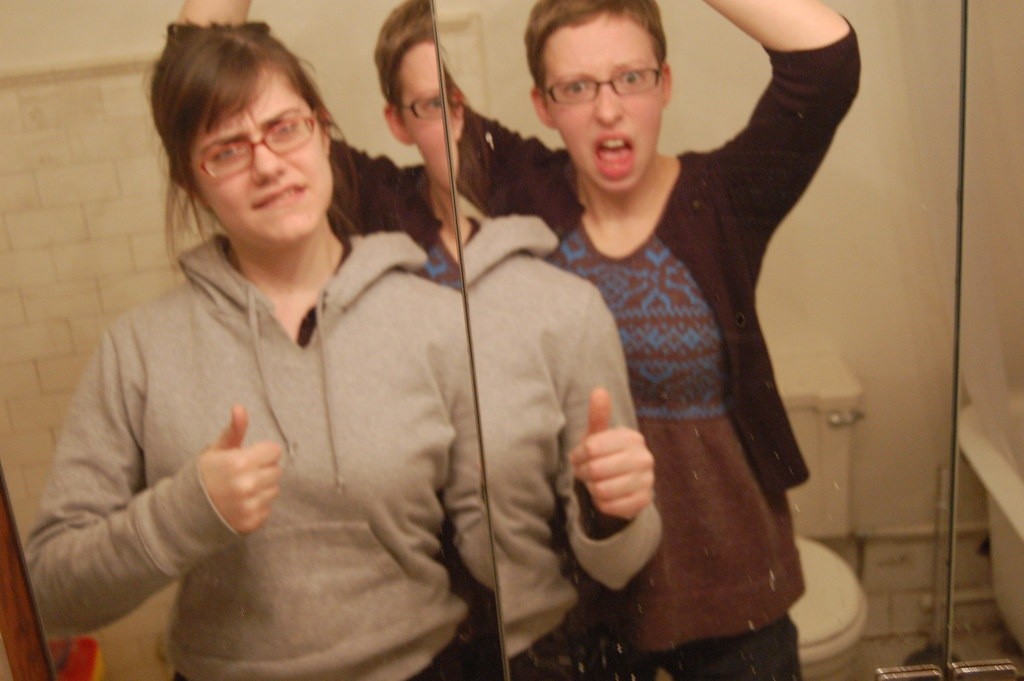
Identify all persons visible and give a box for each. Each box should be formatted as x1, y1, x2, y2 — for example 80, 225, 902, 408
23, 0, 862, 681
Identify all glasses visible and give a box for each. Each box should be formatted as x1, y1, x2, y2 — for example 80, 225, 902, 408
187, 110, 316, 180
539, 66, 662, 105
398, 94, 443, 120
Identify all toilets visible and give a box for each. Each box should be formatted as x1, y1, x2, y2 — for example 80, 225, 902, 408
768, 343, 867, 680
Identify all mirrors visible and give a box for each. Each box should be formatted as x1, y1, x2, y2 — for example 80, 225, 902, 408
0, 0, 1024, 680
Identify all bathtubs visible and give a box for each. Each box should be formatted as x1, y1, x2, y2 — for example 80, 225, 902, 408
957, 390, 1024, 654
